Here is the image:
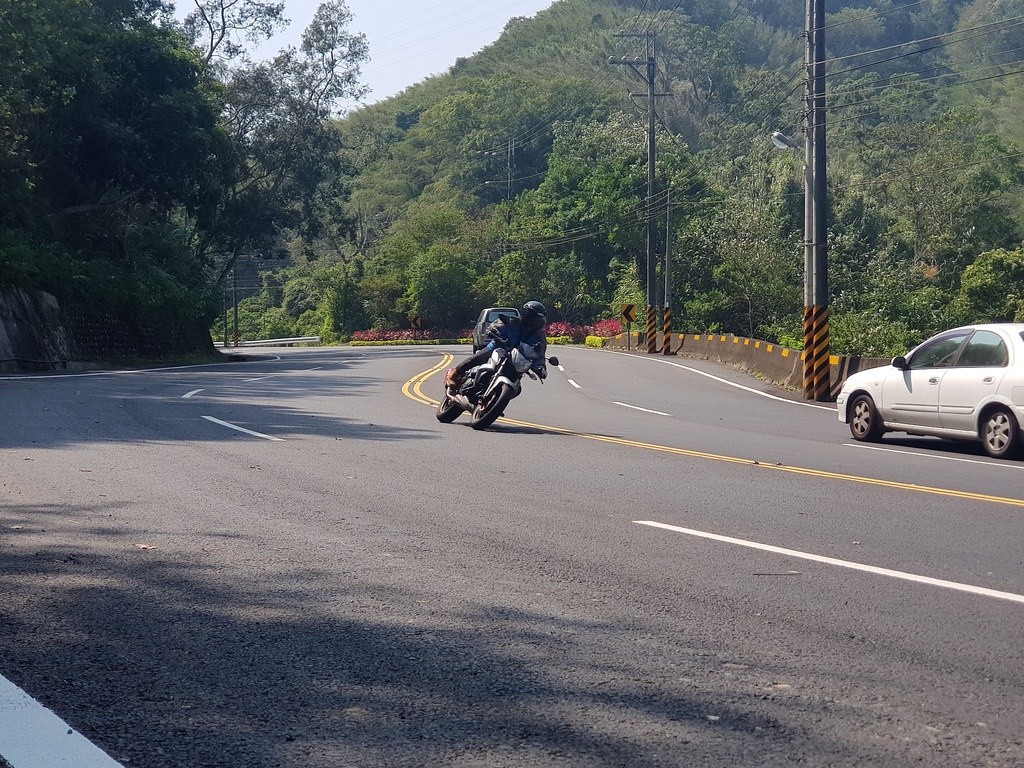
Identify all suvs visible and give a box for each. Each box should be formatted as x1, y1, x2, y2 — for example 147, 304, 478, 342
471, 306, 522, 354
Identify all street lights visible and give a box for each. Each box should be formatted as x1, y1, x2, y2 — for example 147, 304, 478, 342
771, 132, 831, 400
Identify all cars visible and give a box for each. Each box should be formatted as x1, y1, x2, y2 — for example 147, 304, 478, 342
837, 324, 1024, 458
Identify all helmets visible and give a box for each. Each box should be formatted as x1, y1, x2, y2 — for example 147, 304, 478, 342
521, 301, 548, 329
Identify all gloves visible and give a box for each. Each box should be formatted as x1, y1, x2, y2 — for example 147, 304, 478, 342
536, 366, 548, 379
488, 328, 501, 339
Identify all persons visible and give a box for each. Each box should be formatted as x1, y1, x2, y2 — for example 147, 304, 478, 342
446, 300, 548, 388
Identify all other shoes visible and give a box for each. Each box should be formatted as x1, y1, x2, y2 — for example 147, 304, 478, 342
446, 370, 460, 387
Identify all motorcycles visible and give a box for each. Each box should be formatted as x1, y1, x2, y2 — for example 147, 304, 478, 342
436, 313, 559, 430
